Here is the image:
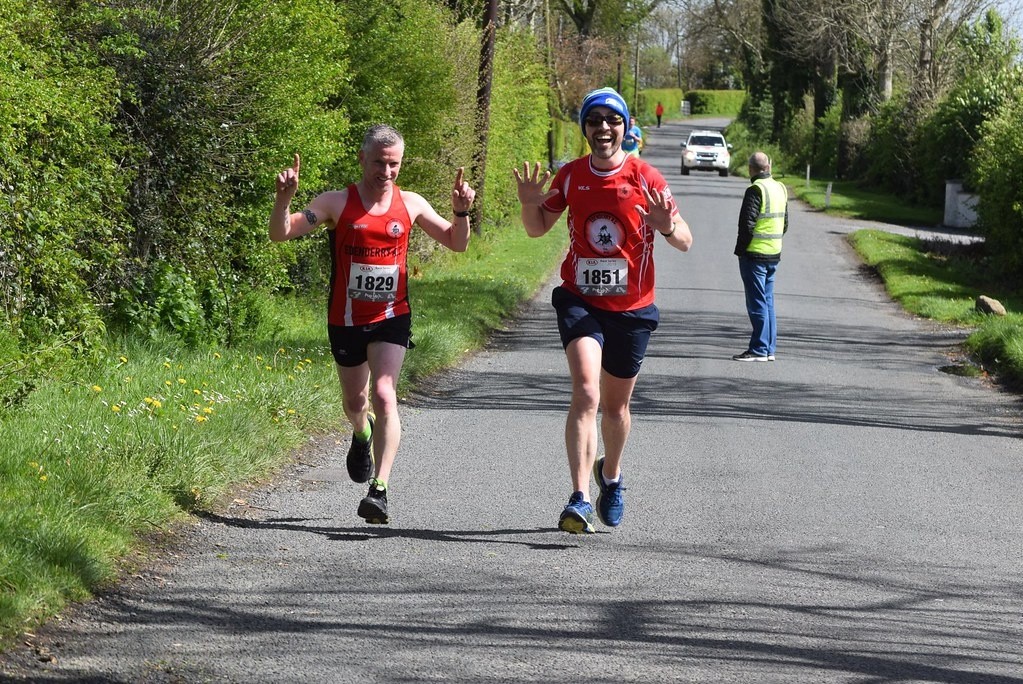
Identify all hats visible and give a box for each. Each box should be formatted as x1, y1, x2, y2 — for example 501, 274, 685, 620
579, 88, 629, 138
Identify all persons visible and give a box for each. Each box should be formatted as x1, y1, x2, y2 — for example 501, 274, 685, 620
733, 152, 788, 362
513, 87, 692, 534
621, 116, 642, 157
270, 125, 476, 525
656, 101, 663, 128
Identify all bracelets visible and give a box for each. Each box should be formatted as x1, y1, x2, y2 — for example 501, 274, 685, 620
453, 208, 469, 217
660, 221, 676, 237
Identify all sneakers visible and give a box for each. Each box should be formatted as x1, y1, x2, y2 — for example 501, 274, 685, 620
592, 455, 626, 526
346, 412, 375, 483
357, 477, 388, 523
559, 491, 595, 536
732, 347, 775, 362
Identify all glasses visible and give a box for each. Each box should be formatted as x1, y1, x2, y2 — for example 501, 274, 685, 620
583, 114, 623, 127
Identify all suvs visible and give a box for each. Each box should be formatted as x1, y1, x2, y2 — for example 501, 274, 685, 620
680, 130, 733, 177
